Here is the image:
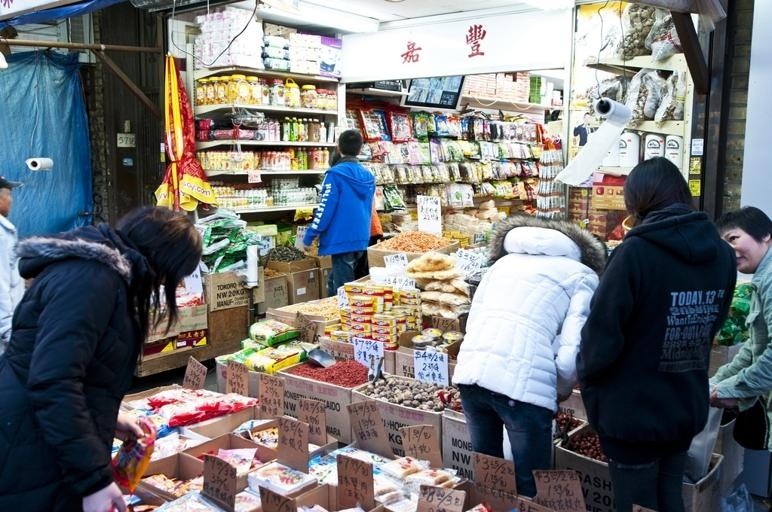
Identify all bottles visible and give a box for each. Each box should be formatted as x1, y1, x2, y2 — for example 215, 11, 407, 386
210, 179, 317, 209
282, 117, 327, 144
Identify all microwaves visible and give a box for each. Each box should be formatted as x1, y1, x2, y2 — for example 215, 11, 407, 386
261, 148, 330, 172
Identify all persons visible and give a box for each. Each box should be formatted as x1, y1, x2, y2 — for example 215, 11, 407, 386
0, 206, 203, 512
301, 131, 374, 295
328, 154, 384, 245
575, 156, 736, 512
450, 214, 609, 496
0, 180, 26, 358
708, 205, 771, 512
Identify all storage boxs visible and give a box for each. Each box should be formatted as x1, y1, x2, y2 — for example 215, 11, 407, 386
112, 228, 753, 511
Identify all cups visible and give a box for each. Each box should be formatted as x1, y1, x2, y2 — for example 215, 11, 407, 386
196, 118, 211, 141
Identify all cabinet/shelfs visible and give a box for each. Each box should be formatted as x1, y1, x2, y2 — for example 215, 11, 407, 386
187, 44, 343, 219
581, 48, 684, 135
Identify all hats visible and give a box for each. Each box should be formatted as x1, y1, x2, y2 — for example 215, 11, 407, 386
0, 176, 21, 189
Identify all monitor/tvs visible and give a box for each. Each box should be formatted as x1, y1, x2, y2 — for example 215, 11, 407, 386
400, 74, 465, 113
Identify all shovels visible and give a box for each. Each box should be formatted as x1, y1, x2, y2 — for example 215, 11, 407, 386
302, 345, 337, 368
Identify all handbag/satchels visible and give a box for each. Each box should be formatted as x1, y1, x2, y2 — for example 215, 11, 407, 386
732, 397, 769, 451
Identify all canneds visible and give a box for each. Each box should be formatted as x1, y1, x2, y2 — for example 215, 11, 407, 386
324, 284, 463, 351
